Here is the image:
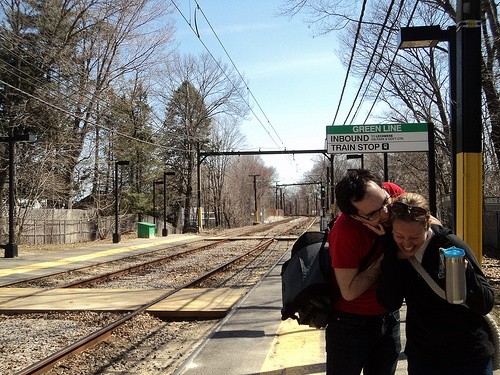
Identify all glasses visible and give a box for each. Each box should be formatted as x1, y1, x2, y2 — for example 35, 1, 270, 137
355, 191, 388, 221
391, 201, 426, 218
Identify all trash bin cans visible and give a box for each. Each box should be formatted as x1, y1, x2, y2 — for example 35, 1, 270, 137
138, 221, 156, 238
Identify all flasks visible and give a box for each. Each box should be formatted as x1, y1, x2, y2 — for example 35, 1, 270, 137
444, 246, 467, 304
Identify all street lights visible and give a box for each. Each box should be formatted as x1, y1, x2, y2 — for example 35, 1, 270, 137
0, 135, 36, 258
112, 160, 129, 242
153, 181, 164, 224
248, 174, 260, 225
163, 170, 176, 237
398, 24, 456, 234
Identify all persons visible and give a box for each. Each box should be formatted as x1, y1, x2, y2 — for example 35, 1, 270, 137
325, 169, 444, 375
362, 193, 496, 375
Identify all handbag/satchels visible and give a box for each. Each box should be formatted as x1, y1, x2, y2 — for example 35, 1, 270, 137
278, 229, 339, 330
479, 313, 500, 374
